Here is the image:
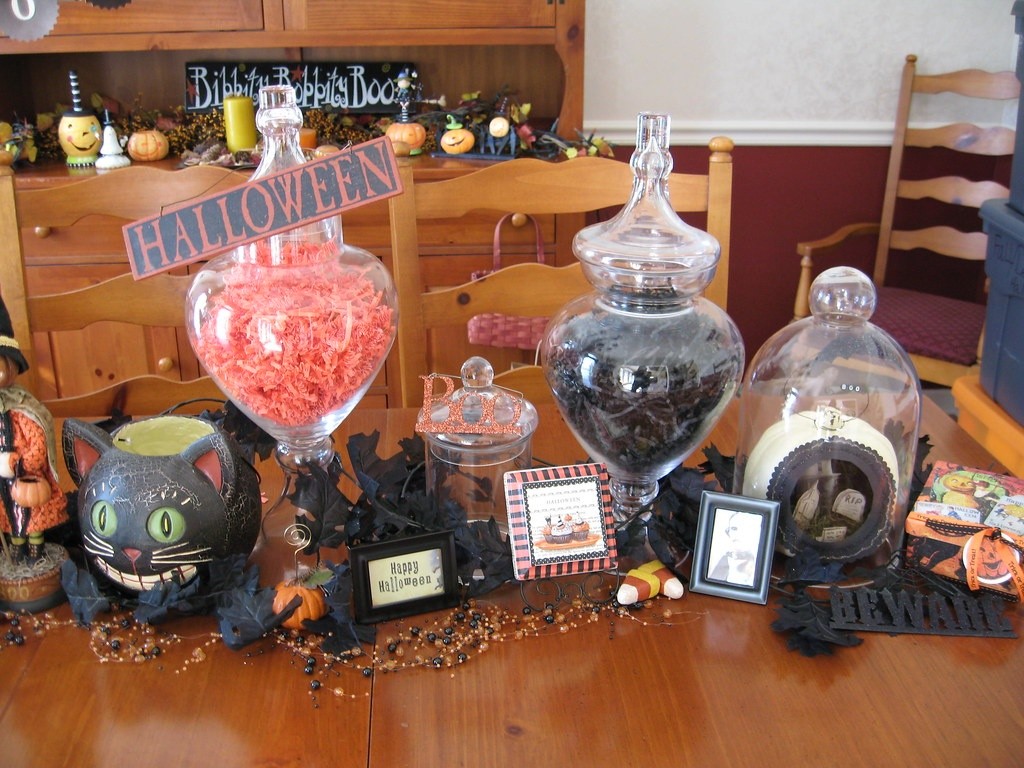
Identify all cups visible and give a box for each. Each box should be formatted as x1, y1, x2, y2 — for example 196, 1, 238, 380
416, 358, 538, 585
222, 97, 257, 156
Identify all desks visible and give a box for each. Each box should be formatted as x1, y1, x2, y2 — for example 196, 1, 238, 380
1, 397, 1024, 768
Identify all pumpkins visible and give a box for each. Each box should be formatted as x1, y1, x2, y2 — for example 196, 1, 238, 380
271, 574, 328, 633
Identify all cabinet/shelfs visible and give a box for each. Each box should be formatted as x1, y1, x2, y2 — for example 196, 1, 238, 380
0, 0, 599, 413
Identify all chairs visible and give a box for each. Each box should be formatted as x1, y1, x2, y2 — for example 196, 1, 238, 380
0, 153, 350, 418
786, 49, 1023, 389
386, 136, 737, 406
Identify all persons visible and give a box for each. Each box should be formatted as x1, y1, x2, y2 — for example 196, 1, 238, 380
393, 67, 423, 102
0, 292, 71, 566
